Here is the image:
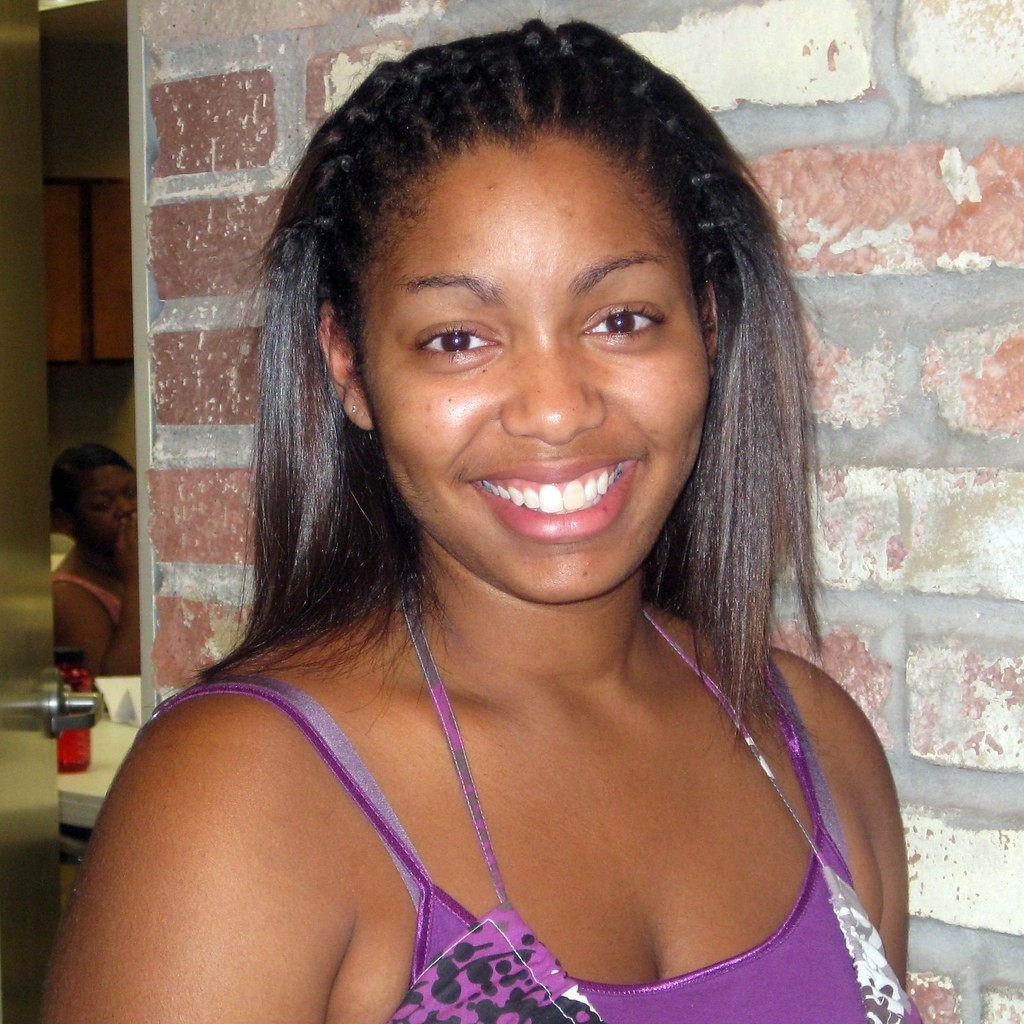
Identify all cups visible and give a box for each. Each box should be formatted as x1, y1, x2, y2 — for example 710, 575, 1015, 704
55, 664, 91, 775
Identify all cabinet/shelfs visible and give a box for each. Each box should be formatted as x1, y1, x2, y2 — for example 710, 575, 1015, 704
42, 175, 135, 363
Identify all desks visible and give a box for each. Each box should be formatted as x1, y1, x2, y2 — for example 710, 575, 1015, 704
57, 717, 141, 826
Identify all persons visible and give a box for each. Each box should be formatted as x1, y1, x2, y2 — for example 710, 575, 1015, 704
35, 19, 917, 1024
49, 438, 137, 679
